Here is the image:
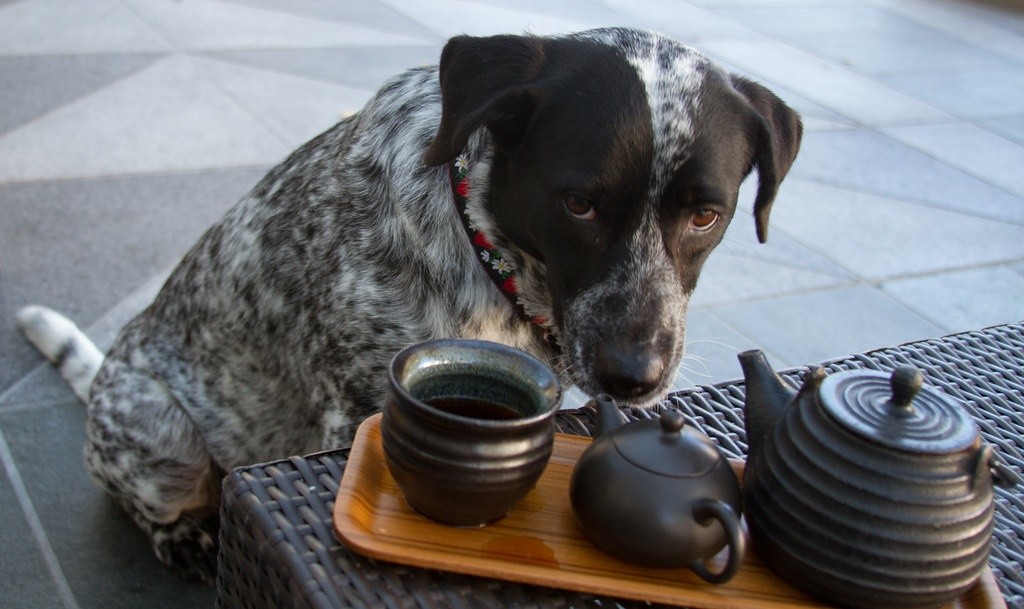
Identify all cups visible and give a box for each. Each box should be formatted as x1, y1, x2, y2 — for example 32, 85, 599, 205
380, 340, 564, 531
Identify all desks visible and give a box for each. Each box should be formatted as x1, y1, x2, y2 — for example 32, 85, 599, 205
217, 323, 1024, 609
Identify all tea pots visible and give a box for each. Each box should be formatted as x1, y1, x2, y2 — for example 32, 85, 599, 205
737, 348, 1018, 609
568, 393, 750, 588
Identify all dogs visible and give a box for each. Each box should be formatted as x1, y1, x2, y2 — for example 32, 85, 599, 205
9, 23, 809, 592
